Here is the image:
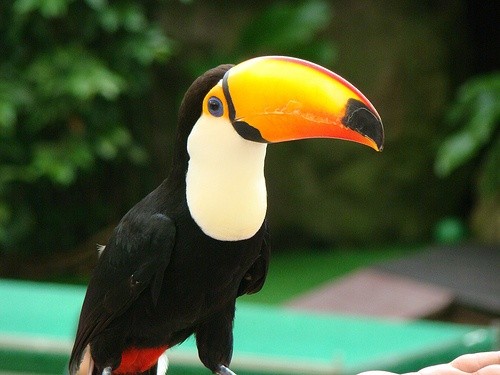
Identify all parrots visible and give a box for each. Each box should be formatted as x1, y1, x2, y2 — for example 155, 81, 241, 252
67, 56, 384, 375
